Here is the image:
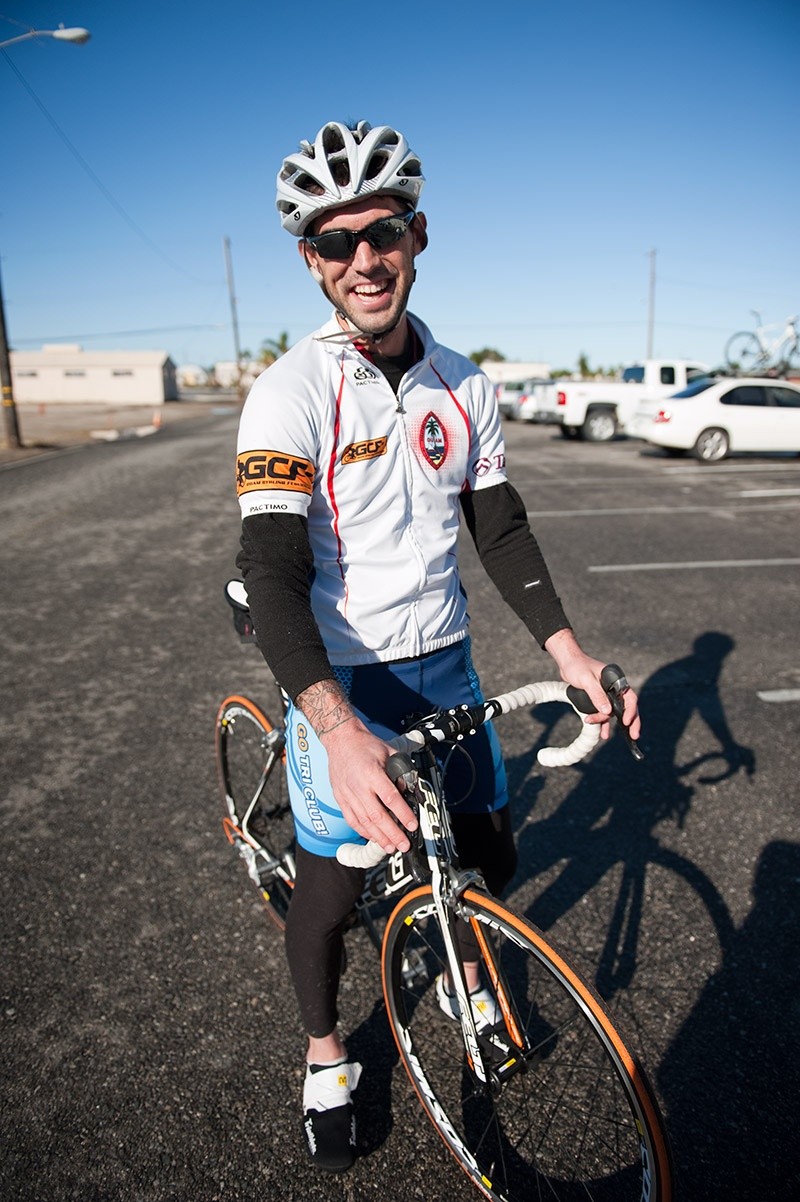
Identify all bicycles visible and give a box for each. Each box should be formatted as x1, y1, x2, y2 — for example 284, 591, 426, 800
210, 682, 677, 1202
723, 308, 800, 375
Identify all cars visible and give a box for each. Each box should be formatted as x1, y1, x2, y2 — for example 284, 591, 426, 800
624, 377, 800, 464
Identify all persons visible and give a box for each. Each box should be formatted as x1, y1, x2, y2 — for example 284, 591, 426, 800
236, 120, 641, 1173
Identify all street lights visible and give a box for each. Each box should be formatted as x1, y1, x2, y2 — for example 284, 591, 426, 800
1, 27, 90, 449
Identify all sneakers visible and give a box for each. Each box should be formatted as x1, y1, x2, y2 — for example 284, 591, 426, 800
433, 973, 532, 1060
303, 1054, 357, 1171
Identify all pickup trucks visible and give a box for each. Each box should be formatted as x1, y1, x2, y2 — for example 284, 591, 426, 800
496, 383, 524, 421
519, 359, 710, 442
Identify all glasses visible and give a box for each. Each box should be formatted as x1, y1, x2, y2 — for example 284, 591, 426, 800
304, 211, 418, 260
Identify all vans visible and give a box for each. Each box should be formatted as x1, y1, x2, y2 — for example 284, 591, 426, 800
480, 361, 549, 384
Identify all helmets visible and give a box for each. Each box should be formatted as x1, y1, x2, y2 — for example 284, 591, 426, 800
275, 120, 424, 236
